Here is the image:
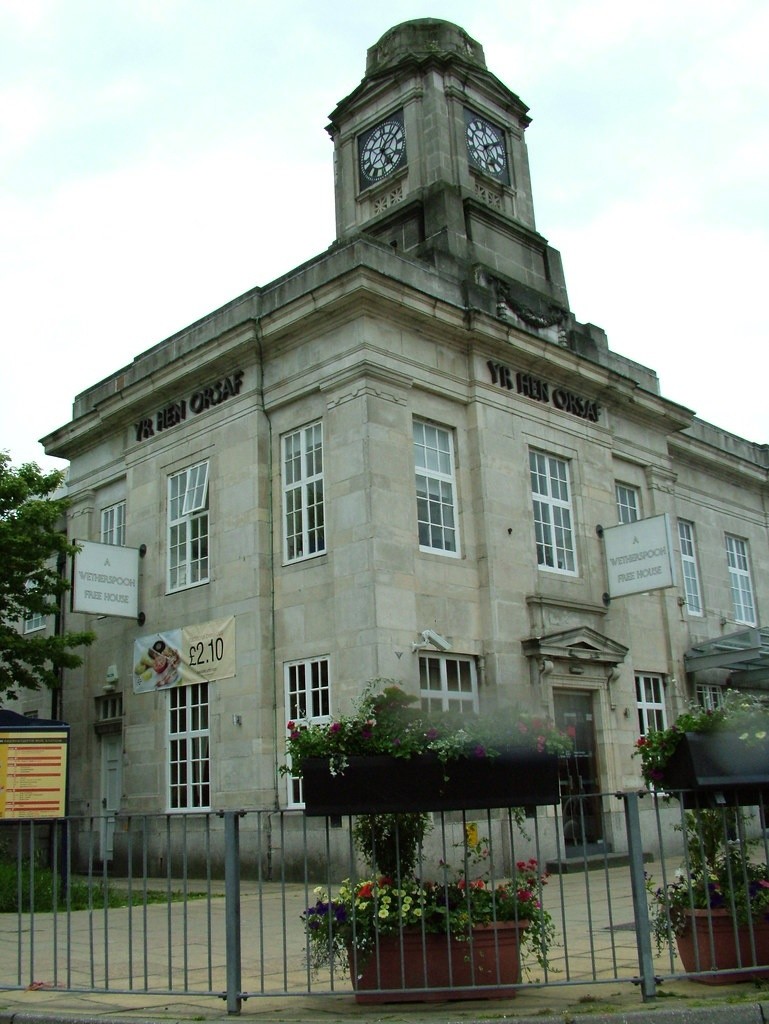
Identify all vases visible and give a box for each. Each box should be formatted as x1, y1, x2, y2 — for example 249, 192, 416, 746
668, 903, 769, 984
302, 744, 560, 819
663, 724, 768, 807
343, 919, 530, 1004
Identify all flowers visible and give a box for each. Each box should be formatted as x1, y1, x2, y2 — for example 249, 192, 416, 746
297, 856, 563, 992
630, 687, 769, 807
275, 675, 577, 838
641, 841, 769, 961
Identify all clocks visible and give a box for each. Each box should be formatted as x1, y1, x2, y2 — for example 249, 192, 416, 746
357, 107, 408, 192
462, 107, 512, 188
